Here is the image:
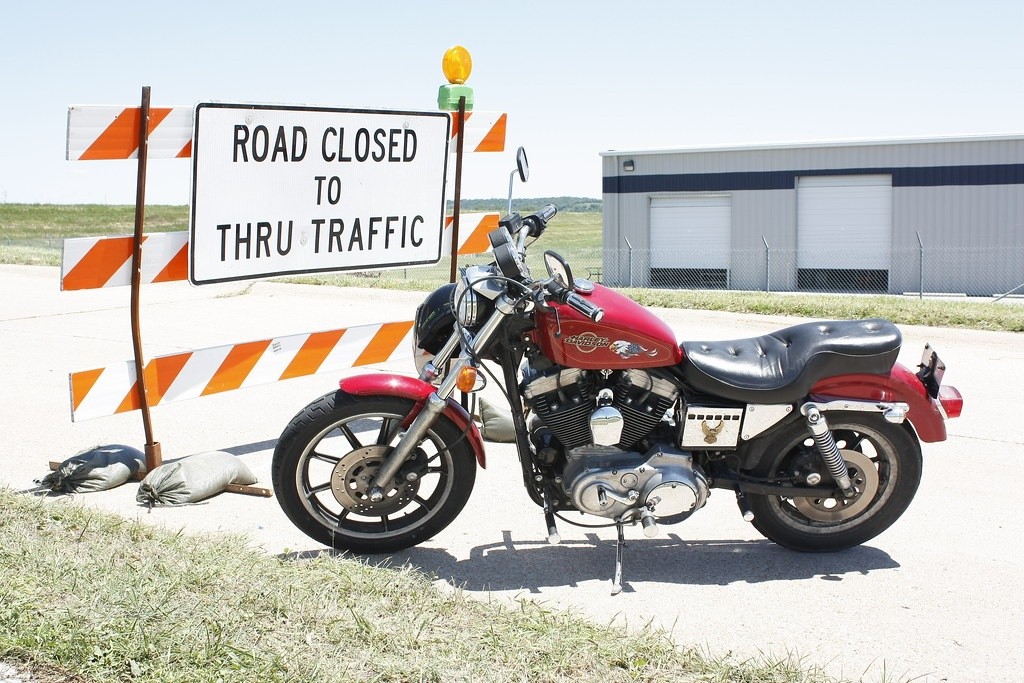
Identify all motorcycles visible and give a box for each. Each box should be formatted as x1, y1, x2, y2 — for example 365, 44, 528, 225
271, 146, 965, 599
